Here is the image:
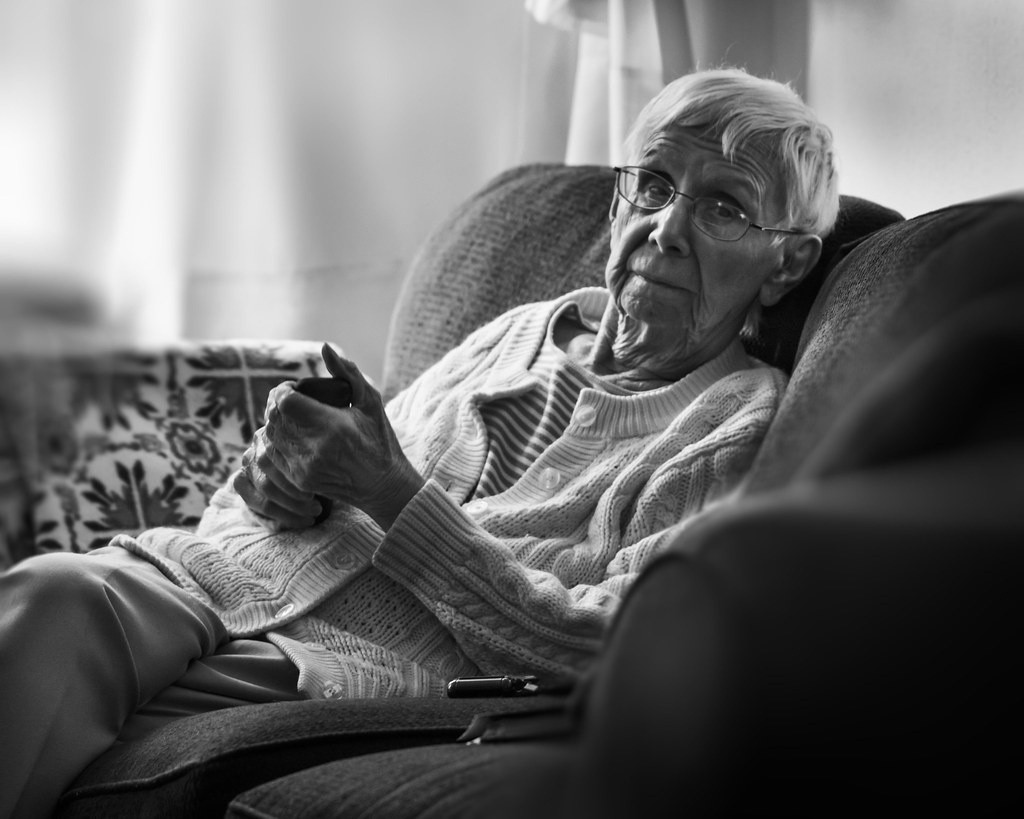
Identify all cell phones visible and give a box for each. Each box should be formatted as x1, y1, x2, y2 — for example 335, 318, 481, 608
294, 377, 352, 409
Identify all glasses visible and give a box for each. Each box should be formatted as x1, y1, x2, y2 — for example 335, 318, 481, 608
613, 166, 798, 242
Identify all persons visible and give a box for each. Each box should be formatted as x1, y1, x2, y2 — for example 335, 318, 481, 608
0, 69, 836, 819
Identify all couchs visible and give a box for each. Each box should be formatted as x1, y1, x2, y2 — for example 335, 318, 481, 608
47, 161, 1024, 819
